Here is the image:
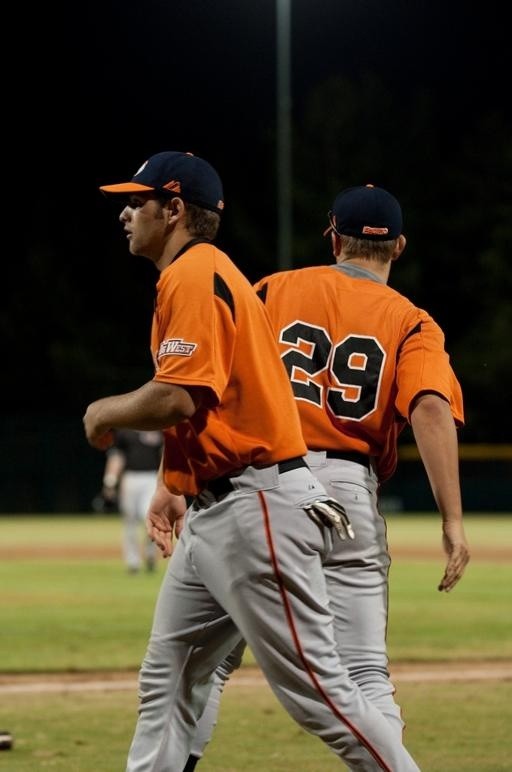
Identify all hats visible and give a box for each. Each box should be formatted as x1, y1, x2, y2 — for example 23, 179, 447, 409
323, 183, 404, 241
99, 150, 223, 210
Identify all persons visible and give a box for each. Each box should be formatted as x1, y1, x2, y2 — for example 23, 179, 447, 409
99, 415, 168, 577
85, 148, 424, 772
180, 183, 470, 772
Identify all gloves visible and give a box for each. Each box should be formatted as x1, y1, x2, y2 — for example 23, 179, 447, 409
304, 499, 354, 540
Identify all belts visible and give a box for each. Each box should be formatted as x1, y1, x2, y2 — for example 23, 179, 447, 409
326, 450, 372, 473
195, 457, 312, 507
